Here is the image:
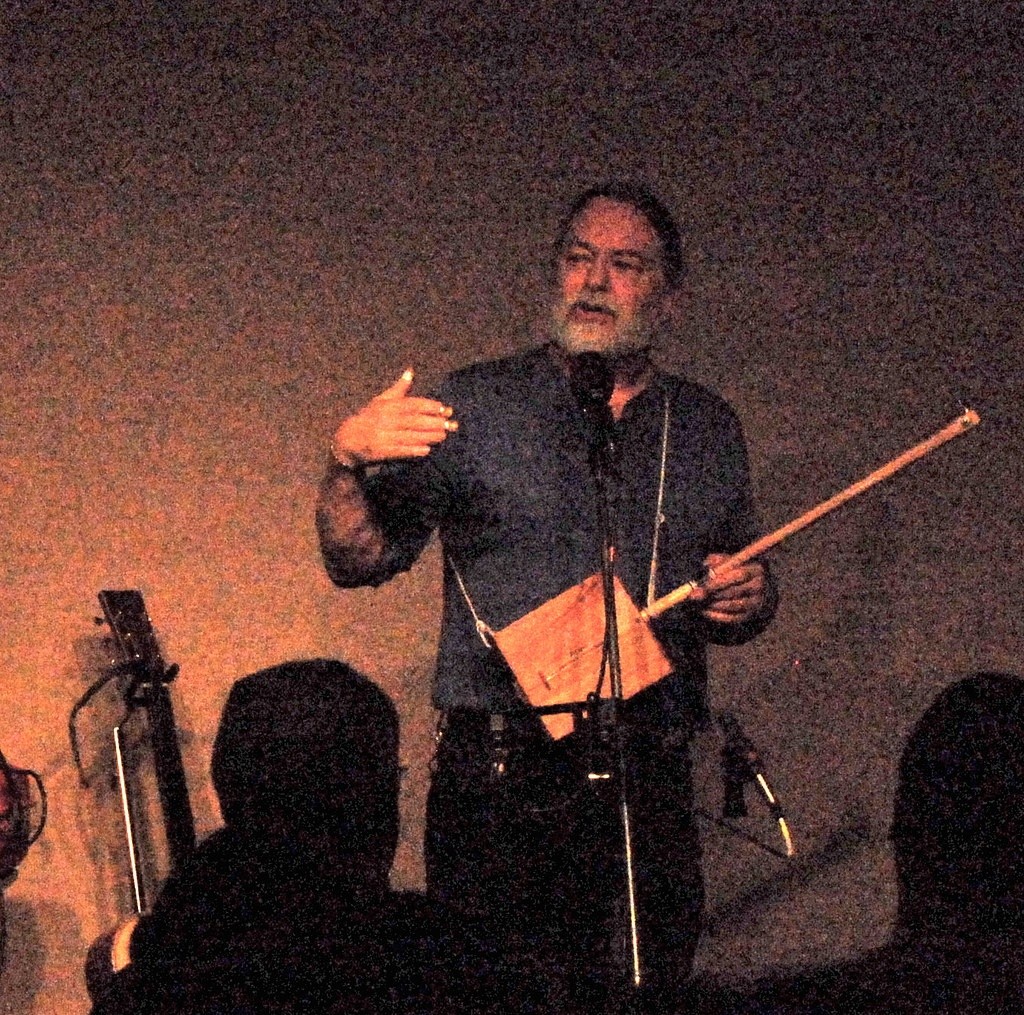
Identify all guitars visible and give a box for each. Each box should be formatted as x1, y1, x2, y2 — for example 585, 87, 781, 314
90, 588, 200, 892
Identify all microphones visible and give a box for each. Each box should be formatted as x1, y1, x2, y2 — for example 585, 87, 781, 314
569, 351, 623, 467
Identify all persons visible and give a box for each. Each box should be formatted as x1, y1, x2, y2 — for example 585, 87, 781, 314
77, 654, 552, 1015
314, 177, 784, 1015
742, 670, 1024, 1015
